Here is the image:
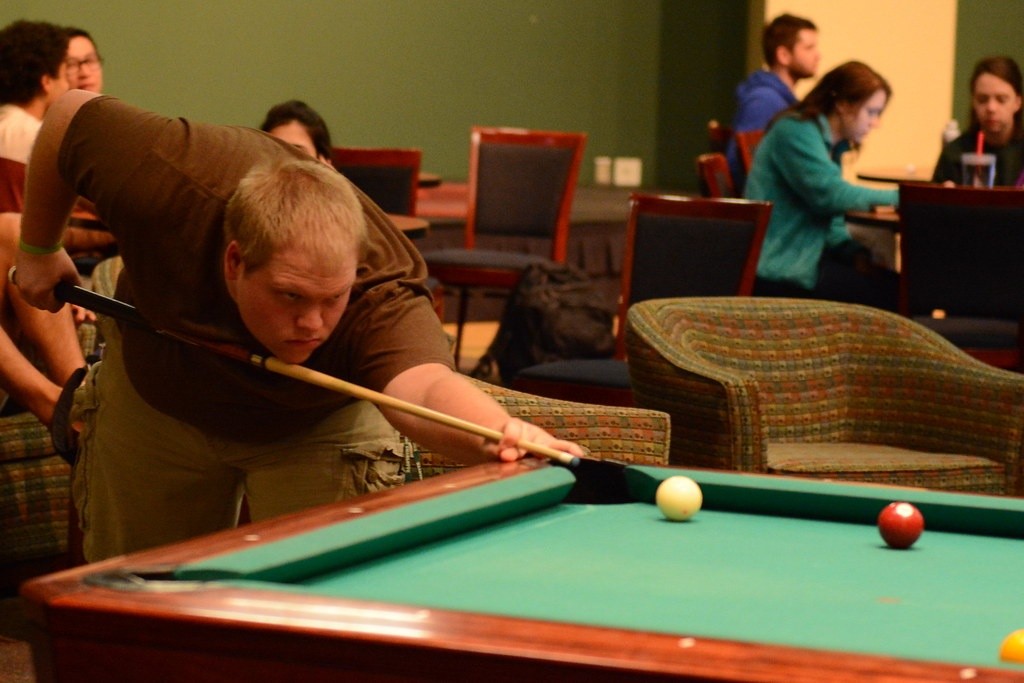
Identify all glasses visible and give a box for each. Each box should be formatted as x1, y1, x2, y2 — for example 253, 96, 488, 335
65, 53, 105, 74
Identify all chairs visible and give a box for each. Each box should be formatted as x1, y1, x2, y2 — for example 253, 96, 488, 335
334, 119, 1024, 508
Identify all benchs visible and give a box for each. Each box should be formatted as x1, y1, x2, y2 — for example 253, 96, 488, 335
0, 257, 673, 643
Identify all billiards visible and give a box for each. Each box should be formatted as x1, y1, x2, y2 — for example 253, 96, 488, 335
655, 474, 704, 522
998, 627, 1024, 665
877, 501, 923, 548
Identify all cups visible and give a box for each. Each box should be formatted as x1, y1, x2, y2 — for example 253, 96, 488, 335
961, 154, 996, 187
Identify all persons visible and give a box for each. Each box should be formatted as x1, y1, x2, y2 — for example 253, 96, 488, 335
744, 61, 900, 310
932, 57, 1024, 187
0, 20, 103, 433
14, 88, 585, 563
727, 14, 821, 174
262, 99, 335, 166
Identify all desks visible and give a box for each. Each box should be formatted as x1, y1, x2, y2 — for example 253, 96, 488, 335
422, 175, 701, 276
858, 168, 932, 183
846, 210, 908, 318
18, 456, 1024, 683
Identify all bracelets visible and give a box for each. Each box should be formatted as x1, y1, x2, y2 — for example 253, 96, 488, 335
20, 239, 63, 254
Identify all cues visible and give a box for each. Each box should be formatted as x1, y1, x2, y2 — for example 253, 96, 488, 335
10, 263, 584, 468
